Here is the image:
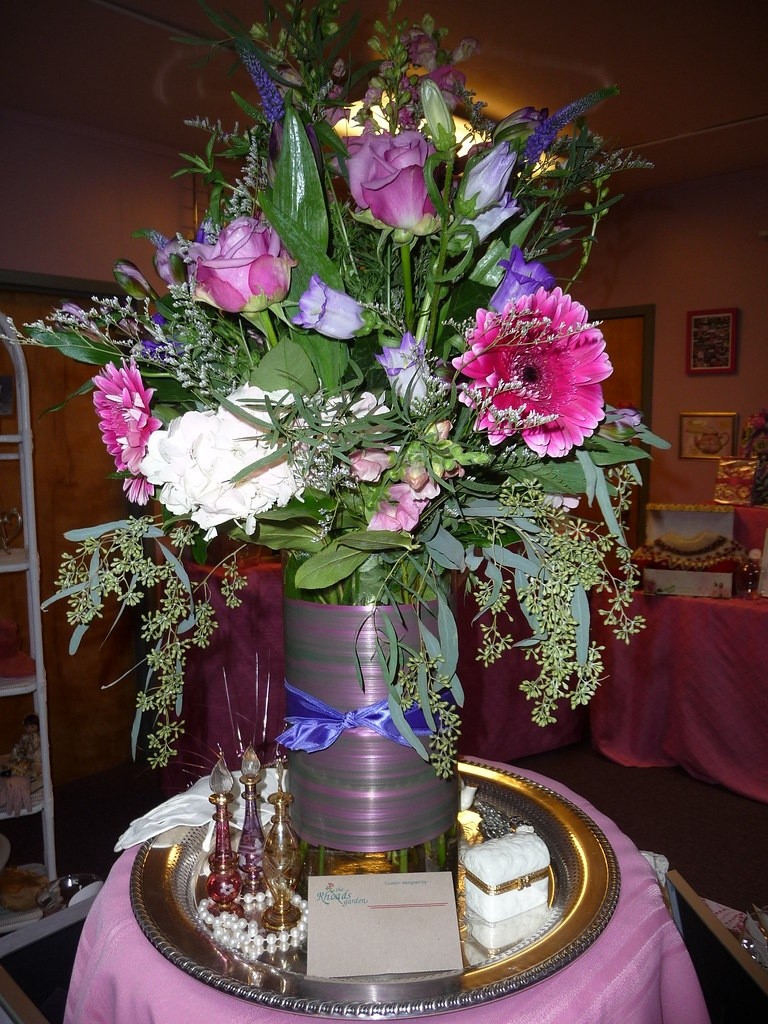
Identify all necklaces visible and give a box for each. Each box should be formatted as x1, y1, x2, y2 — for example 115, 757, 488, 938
198, 888, 309, 959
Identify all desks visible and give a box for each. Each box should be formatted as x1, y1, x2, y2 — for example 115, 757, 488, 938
583, 585, 766, 803
153, 558, 580, 763
63, 756, 709, 1023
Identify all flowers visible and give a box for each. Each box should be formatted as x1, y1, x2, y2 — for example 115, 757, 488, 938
0, 1, 673, 894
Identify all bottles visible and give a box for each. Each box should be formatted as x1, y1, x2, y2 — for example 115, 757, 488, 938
739, 557, 759, 600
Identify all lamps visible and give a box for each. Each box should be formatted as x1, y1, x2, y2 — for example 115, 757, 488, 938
261, 751, 303, 932
206, 742, 244, 918
237, 742, 266, 895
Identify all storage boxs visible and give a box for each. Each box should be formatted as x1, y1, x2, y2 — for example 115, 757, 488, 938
464, 831, 550, 923
643, 501, 733, 599
714, 455, 768, 506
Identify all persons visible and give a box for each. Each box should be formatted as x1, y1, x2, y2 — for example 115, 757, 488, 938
17, 712, 43, 776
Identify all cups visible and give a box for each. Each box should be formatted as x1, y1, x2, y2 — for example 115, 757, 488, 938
739, 910, 768, 970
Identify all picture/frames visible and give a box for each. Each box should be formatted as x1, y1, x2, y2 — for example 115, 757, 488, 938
686, 308, 736, 376
678, 412, 737, 462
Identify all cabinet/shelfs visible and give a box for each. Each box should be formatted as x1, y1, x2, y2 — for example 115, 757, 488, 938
0, 313, 60, 937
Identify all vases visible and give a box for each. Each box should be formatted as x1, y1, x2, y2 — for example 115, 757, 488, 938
283, 552, 460, 895
